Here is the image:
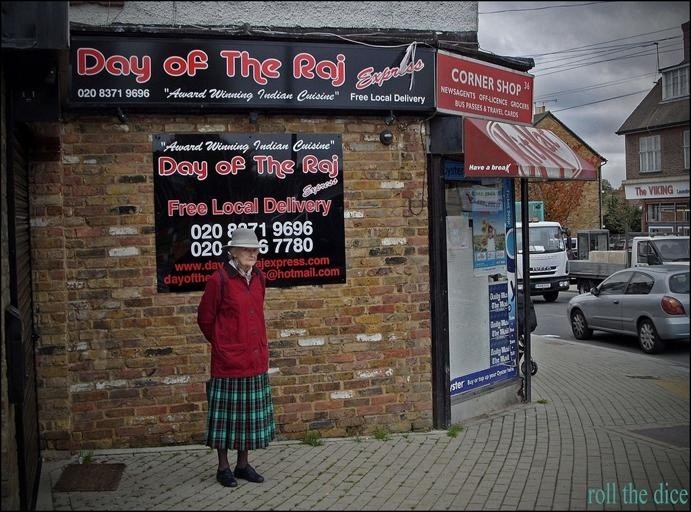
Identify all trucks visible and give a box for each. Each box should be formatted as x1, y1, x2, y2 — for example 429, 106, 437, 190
515, 221, 690, 301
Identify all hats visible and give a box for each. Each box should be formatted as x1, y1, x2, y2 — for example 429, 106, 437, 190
221, 228, 266, 251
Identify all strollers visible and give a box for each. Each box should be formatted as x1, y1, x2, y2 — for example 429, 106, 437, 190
518, 290, 537, 376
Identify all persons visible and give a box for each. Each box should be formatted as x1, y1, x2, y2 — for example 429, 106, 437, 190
484, 220, 498, 253
196, 227, 277, 489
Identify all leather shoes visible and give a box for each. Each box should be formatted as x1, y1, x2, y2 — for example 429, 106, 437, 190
215, 467, 238, 488
234, 464, 265, 484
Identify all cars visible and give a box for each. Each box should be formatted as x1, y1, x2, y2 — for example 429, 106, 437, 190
566, 264, 689, 354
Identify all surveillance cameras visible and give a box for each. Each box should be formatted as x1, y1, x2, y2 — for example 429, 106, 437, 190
381, 130, 393, 144
